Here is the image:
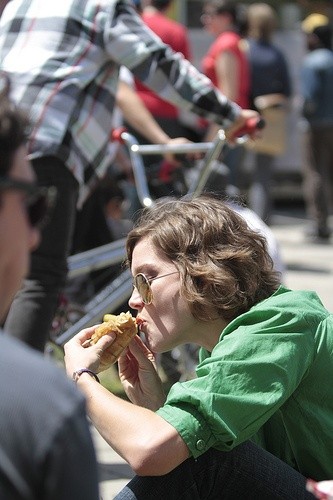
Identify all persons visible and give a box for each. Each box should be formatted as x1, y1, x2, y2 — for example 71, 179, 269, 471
0, 97, 104, 499
243, 3, 289, 225
105, 62, 204, 167
292, 12, 333, 241
0, 0, 262, 357
201, 1, 270, 222
62, 195, 333, 499
121, 0, 190, 184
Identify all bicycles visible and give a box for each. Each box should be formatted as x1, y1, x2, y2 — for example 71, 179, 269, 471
41, 116, 268, 381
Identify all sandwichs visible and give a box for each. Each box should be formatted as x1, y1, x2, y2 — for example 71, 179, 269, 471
89, 311, 138, 374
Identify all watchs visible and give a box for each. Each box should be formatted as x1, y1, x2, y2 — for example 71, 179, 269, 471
72, 368, 100, 384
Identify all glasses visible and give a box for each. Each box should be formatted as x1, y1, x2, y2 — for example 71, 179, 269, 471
131, 270, 181, 305
0, 174, 57, 225
